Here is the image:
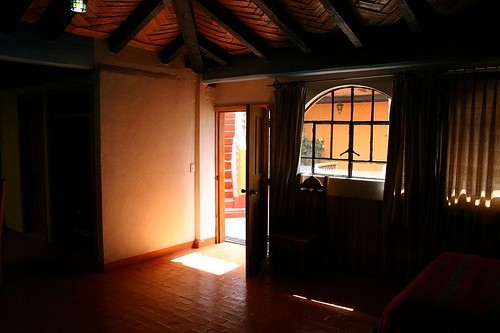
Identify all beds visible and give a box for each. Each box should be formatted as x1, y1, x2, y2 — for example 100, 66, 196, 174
381, 249, 500, 333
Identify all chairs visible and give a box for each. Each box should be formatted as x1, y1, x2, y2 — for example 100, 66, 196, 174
272, 173, 328, 280
0, 178, 8, 235
